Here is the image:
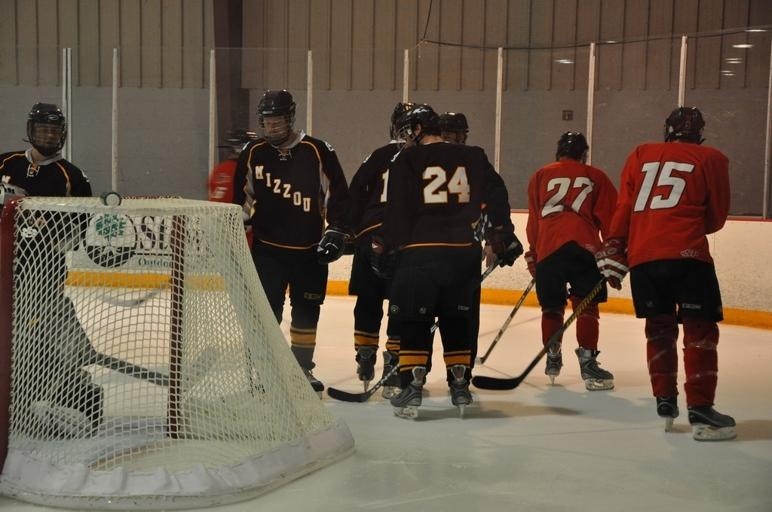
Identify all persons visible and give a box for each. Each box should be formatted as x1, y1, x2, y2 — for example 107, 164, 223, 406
525, 129, 618, 382
205, 129, 258, 261
343, 103, 455, 389
438, 113, 469, 145
365, 103, 523, 407
1, 102, 104, 434
232, 90, 353, 389
607, 107, 736, 429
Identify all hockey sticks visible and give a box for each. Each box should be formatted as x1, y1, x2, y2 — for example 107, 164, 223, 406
327, 242, 516, 402
96, 222, 249, 308
95, 349, 215, 391
472, 275, 607, 390
475, 275, 536, 364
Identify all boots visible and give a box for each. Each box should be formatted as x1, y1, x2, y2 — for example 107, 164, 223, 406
381, 351, 426, 408
545, 342, 562, 375
355, 346, 376, 381
449, 365, 472, 405
575, 347, 614, 380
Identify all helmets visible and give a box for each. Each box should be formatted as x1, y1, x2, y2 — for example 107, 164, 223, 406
257, 91, 296, 145
26, 103, 66, 156
556, 131, 588, 163
665, 106, 706, 145
391, 102, 468, 149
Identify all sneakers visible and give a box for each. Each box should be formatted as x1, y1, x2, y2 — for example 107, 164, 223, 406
688, 406, 736, 428
300, 360, 324, 392
656, 395, 679, 418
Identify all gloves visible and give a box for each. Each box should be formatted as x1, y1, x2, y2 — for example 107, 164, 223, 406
594, 237, 630, 290
492, 234, 523, 267
524, 252, 537, 278
316, 227, 352, 263
